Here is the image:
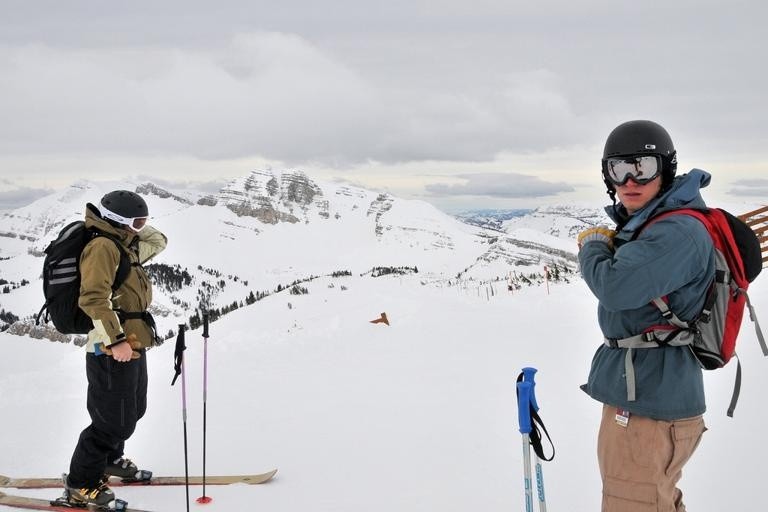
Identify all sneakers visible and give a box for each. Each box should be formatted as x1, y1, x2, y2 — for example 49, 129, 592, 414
104, 455, 137, 477
77, 479, 114, 505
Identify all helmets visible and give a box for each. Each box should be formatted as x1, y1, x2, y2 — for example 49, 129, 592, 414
101, 191, 148, 230
602, 120, 674, 199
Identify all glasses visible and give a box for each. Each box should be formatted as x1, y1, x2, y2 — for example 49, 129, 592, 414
97, 198, 147, 232
602, 151, 677, 186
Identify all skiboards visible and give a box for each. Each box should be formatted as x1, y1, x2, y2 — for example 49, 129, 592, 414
0, 469, 278, 511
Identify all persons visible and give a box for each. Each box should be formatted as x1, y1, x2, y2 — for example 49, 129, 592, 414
34, 189, 167, 510
577, 120, 767, 512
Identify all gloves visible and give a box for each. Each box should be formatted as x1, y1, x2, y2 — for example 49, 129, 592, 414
94, 333, 142, 359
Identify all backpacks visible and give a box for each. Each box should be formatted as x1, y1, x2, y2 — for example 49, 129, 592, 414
604, 209, 768, 417
36, 221, 160, 344
